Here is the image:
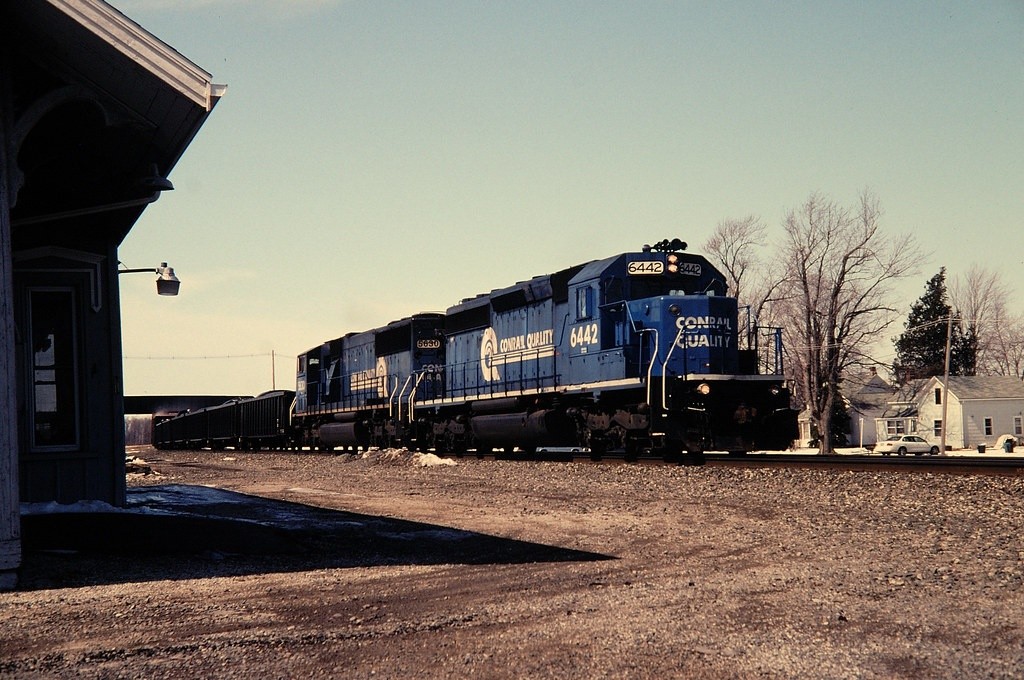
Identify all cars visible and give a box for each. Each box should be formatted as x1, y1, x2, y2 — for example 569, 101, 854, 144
810, 435, 838, 447
875, 435, 941, 455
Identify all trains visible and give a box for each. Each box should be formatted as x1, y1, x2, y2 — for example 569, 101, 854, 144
152, 237, 802, 457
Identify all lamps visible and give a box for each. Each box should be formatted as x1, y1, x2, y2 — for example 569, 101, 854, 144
118, 262, 181, 296
141, 162, 173, 192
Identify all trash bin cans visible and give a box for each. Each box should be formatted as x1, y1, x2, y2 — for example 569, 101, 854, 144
1005, 439, 1014, 452
977, 445, 985, 453
